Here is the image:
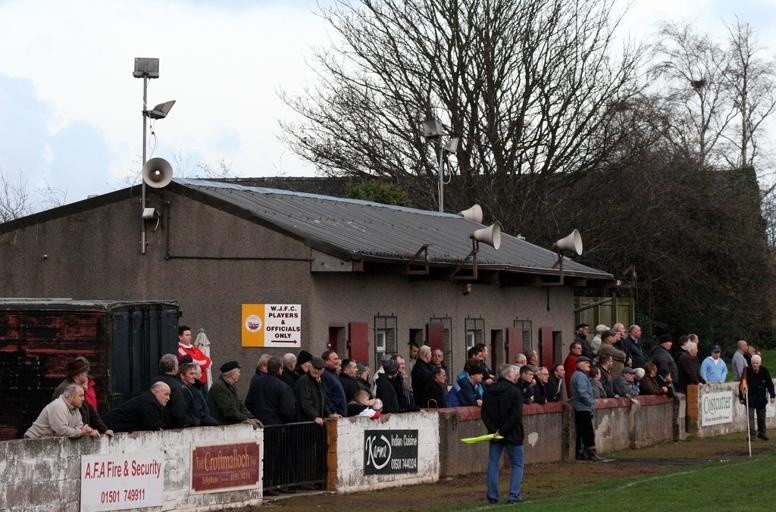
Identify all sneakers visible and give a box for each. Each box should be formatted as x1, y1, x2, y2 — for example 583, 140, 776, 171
758, 434, 769, 440
747, 436, 756, 440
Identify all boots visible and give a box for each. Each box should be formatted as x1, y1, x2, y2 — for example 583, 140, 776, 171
576, 446, 605, 461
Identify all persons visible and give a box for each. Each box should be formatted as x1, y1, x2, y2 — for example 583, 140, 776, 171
564, 324, 712, 432
732, 341, 756, 381
480, 365, 524, 504
102, 354, 264, 430
245, 350, 348, 493
321, 343, 495, 423
738, 354, 774, 441
700, 345, 728, 386
511, 349, 566, 404
570, 357, 606, 462
25, 356, 114, 438
177, 326, 211, 382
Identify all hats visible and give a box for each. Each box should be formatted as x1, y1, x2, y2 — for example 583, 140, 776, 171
659, 334, 672, 344
623, 367, 636, 373
576, 356, 592, 363
711, 345, 721, 352
470, 364, 482, 375
220, 361, 242, 373
64, 359, 89, 378
381, 358, 400, 376
311, 357, 326, 369
576, 324, 616, 339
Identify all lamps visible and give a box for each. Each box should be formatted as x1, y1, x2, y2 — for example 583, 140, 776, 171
133, 58, 159, 78
443, 137, 460, 154
143, 100, 177, 119
422, 117, 444, 137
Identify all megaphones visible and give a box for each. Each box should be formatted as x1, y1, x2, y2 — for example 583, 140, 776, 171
557, 229, 583, 256
143, 157, 174, 190
461, 204, 482, 223
474, 223, 502, 250
